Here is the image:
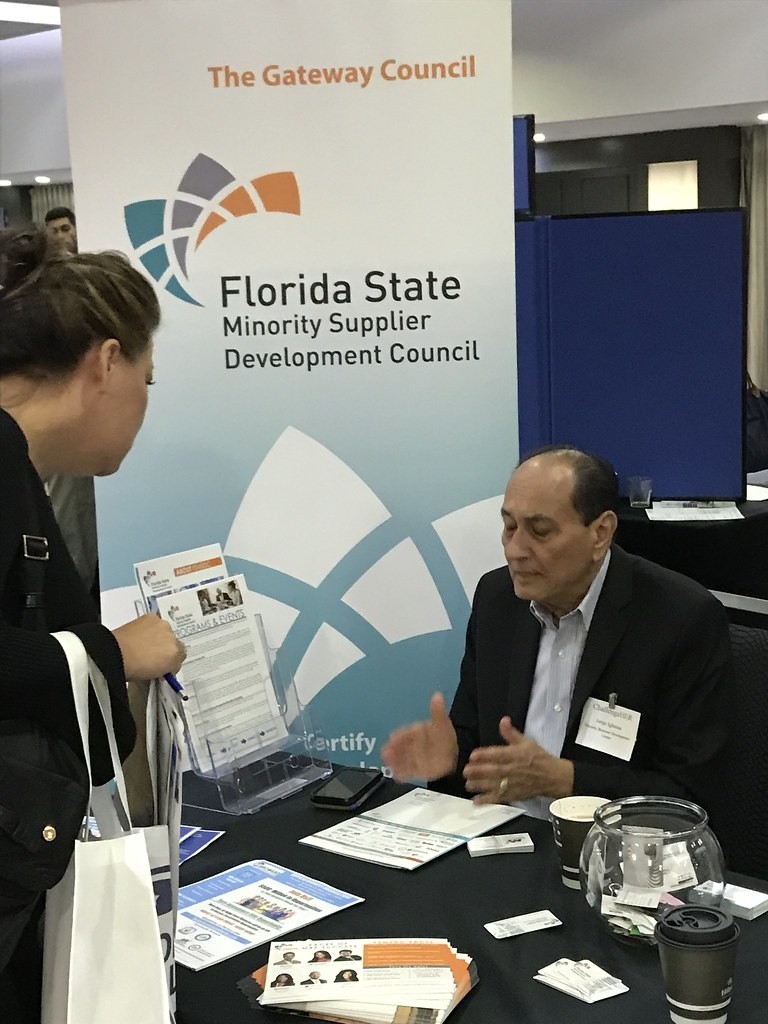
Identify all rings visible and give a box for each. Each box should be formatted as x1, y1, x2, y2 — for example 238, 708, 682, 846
495, 777, 509, 797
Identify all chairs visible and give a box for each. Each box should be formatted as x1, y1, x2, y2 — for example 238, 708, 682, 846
701, 587, 768, 883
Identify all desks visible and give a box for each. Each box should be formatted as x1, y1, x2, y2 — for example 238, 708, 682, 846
176, 750, 768, 1024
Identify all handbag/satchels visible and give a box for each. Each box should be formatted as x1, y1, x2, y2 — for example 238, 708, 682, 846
41, 631, 171, 1024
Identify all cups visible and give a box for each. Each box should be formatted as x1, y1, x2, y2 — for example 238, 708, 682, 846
626, 477, 653, 507
655, 904, 741, 1024
549, 796, 622, 889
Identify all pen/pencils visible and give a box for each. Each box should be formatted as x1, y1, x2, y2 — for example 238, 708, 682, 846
163, 672, 190, 701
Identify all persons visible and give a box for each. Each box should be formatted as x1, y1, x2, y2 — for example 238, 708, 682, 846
0, 217, 188, 1024
44, 208, 77, 255
381, 447, 735, 830
241, 893, 361, 990
200, 581, 243, 614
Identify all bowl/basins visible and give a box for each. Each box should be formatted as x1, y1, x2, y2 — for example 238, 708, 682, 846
579, 796, 727, 947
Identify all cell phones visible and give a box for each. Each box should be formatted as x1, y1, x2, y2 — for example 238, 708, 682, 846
309, 767, 384, 807
